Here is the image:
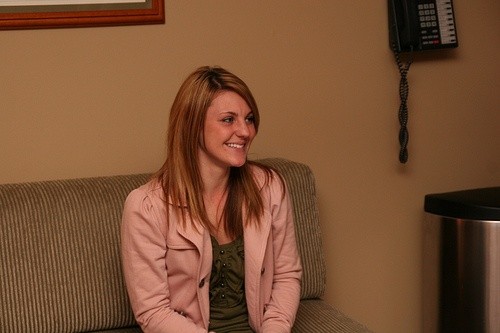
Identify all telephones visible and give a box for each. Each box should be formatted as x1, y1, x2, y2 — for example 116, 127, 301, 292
388, 0, 459, 54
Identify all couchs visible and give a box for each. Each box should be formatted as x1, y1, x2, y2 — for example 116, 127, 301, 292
0, 155, 375, 333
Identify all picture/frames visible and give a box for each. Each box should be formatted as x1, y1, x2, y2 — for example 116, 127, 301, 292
0, 1, 165, 31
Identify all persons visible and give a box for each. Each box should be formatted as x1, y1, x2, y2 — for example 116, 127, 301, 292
118, 63, 304, 333
